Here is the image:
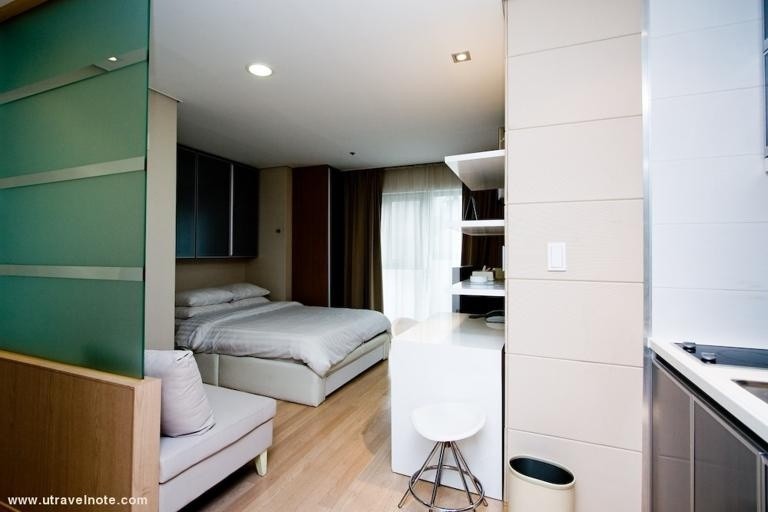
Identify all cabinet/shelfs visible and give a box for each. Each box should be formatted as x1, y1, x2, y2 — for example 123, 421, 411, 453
292, 164, 346, 308
649, 355, 768, 512
443, 150, 506, 297
177, 143, 230, 259
231, 160, 259, 258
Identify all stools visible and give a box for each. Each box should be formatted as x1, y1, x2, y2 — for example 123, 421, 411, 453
399, 403, 488, 512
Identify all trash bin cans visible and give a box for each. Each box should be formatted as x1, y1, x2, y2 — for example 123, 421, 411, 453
507, 454, 576, 512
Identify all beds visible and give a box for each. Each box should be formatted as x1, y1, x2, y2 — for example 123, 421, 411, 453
174, 301, 392, 407
158, 383, 276, 512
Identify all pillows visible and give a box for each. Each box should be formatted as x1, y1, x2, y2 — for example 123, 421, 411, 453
231, 297, 271, 310
176, 287, 235, 307
215, 280, 271, 302
175, 302, 233, 319
143, 347, 214, 437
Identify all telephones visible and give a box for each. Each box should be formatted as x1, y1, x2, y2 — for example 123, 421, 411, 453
485, 310, 505, 322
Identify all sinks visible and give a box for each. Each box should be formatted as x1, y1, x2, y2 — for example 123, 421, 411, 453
728, 379, 768, 405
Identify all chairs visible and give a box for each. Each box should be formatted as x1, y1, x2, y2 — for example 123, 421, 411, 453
391, 318, 418, 336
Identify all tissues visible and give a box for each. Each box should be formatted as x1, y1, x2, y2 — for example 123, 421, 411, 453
472, 264, 494, 281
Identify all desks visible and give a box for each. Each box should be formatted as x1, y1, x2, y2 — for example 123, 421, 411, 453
390, 312, 504, 501
